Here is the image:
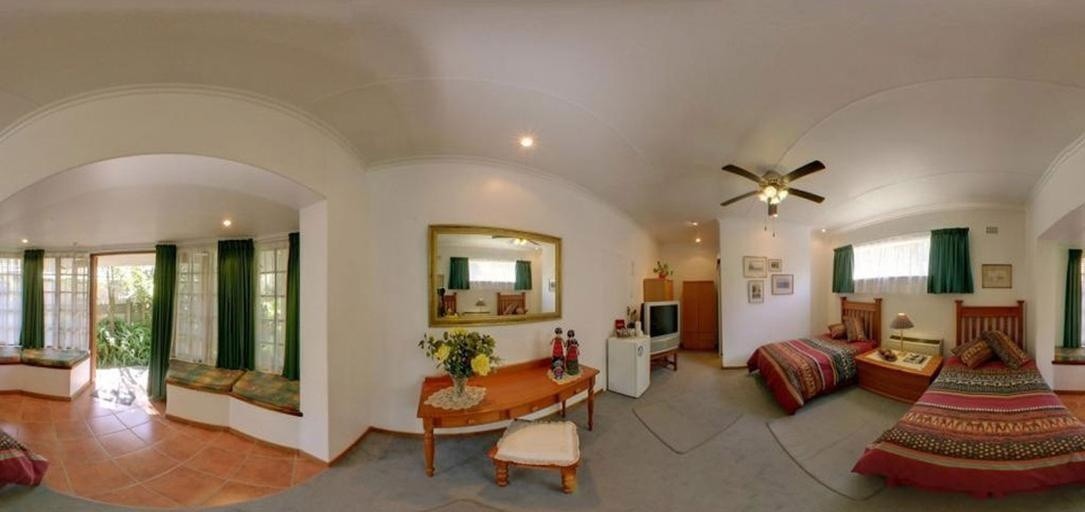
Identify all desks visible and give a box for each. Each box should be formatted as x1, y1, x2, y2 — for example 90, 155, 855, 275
416, 358, 600, 477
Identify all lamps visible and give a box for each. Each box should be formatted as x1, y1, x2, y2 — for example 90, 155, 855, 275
475, 298, 486, 312
889, 313, 915, 351
759, 181, 788, 236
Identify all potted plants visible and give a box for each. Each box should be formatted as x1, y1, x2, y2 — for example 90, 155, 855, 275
653, 261, 673, 279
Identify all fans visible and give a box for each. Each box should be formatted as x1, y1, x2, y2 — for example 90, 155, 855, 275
720, 160, 825, 206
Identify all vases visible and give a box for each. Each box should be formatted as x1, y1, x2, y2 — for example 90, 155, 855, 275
450, 374, 468, 400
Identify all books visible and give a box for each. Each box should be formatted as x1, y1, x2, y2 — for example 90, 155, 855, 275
904, 353, 926, 365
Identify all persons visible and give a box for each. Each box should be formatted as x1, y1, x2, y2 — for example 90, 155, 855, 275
565, 330, 581, 374
549, 327, 565, 379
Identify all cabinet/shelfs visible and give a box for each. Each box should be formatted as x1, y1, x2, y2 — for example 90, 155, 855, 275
682, 281, 717, 351
644, 278, 674, 302
651, 347, 678, 371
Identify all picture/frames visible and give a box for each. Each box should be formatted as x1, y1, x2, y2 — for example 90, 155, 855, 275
743, 256, 793, 304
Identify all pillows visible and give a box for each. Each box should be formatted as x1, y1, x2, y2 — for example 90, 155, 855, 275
950, 336, 994, 369
504, 302, 528, 315
842, 315, 870, 343
828, 324, 847, 339
981, 330, 1032, 369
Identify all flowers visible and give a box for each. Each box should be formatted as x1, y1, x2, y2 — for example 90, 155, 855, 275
417, 328, 505, 393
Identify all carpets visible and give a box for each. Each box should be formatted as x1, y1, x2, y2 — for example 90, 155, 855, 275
0, 348, 1085, 512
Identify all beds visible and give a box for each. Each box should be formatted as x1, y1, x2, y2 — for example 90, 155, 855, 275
850, 300, 1085, 499
746, 296, 883, 415
496, 292, 531, 316
443, 293, 458, 317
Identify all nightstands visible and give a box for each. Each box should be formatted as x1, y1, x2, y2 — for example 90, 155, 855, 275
853, 348, 943, 404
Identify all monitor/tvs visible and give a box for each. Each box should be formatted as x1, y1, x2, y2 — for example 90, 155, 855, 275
640, 301, 681, 353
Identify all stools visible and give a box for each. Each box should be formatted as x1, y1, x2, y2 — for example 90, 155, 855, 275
485, 417, 582, 494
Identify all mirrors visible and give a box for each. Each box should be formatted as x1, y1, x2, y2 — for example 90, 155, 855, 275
427, 225, 562, 326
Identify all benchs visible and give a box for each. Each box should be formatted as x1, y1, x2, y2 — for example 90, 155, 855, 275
164, 359, 301, 451
0, 344, 91, 401
